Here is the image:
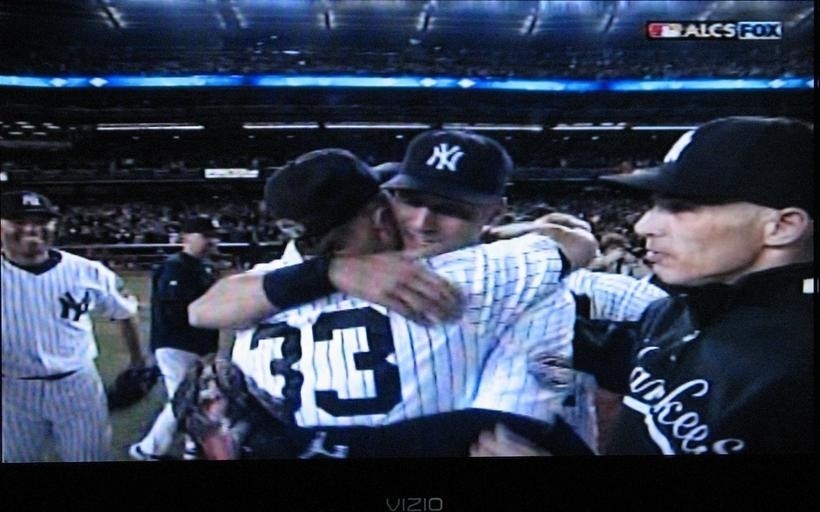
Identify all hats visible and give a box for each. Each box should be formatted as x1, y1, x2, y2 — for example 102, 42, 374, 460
265, 146, 381, 240
598, 117, 819, 220
1, 191, 62, 222
182, 214, 229, 236
379, 129, 512, 205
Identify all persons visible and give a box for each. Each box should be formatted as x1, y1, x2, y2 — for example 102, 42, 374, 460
2, 103, 820, 462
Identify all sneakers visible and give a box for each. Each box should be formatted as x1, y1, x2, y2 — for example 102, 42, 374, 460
128, 444, 171, 462
182, 448, 203, 461
